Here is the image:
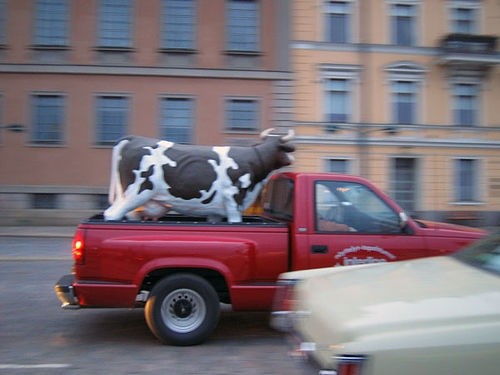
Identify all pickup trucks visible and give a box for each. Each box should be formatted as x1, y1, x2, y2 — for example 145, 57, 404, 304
56, 172, 490, 346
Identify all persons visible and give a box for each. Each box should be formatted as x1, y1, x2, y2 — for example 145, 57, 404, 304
318, 220, 357, 232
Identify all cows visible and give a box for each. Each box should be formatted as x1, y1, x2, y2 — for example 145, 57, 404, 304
102, 127, 297, 223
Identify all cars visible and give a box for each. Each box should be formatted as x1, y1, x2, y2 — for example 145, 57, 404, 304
271, 230, 500, 375
